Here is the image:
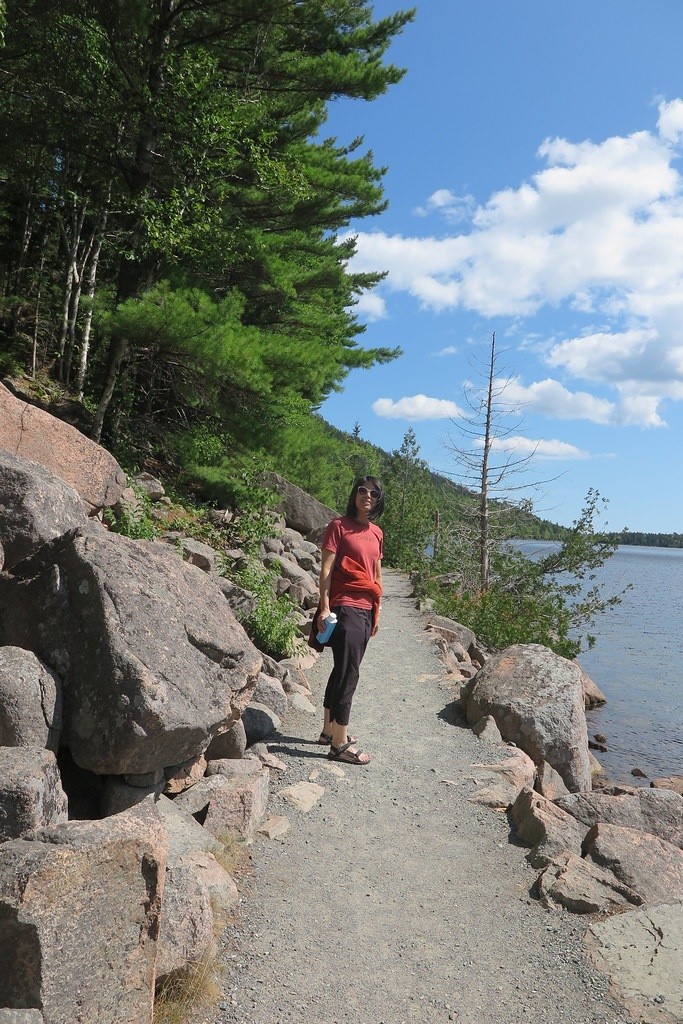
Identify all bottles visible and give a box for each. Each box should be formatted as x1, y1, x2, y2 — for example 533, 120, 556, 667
316, 613, 338, 644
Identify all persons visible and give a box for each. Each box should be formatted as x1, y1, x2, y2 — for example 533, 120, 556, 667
317, 475, 385, 765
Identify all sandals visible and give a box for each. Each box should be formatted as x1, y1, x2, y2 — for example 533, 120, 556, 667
319, 733, 356, 745
327, 743, 371, 765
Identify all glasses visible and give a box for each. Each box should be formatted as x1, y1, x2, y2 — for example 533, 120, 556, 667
358, 486, 380, 498
